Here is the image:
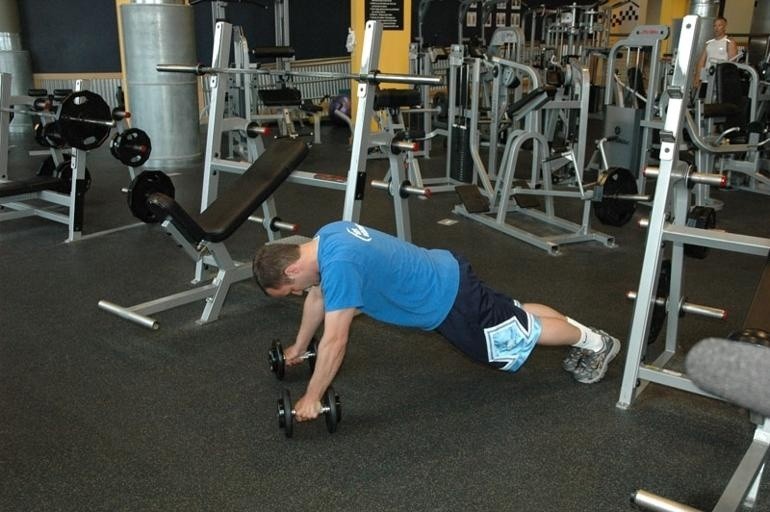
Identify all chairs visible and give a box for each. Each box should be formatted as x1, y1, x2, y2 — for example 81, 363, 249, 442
574, 336, 621, 384
560, 326, 609, 372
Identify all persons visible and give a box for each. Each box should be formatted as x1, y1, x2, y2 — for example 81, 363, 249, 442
255, 220, 622, 423
692, 17, 738, 100
